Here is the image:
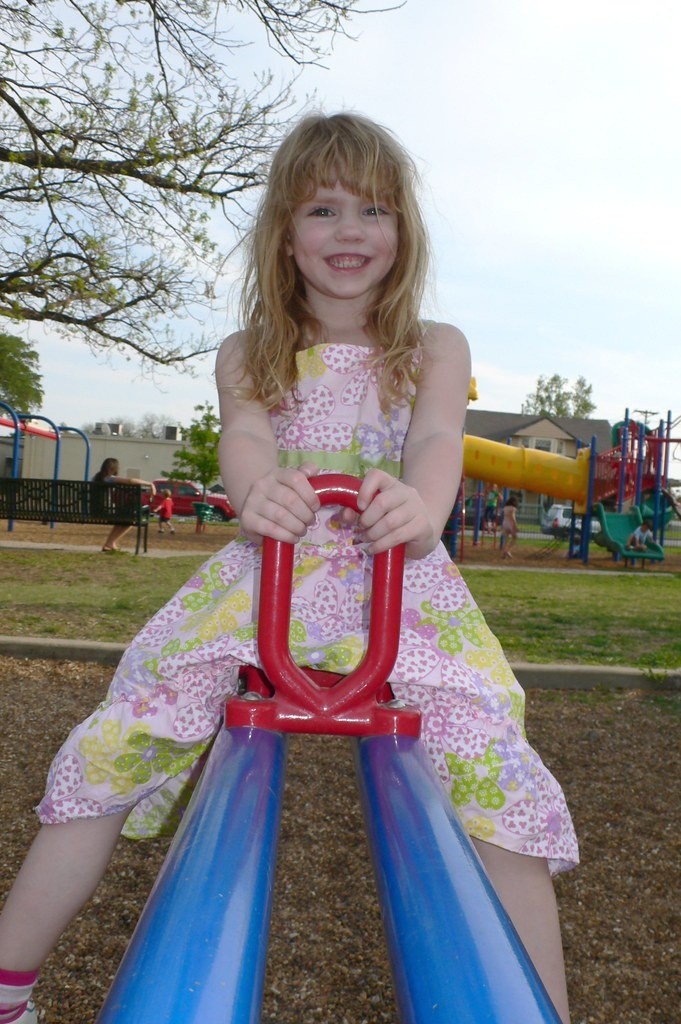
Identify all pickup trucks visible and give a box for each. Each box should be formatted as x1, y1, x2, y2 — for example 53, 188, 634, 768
112, 478, 237, 524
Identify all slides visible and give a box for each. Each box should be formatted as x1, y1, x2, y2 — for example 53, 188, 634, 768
593, 497, 674, 562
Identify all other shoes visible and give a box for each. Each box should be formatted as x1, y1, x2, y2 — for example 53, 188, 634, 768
170, 530, 176, 534
0, 999, 45, 1024
505, 552, 513, 559
159, 529, 164, 533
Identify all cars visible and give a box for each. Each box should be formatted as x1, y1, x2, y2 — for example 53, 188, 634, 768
460, 490, 505, 533
541, 504, 603, 543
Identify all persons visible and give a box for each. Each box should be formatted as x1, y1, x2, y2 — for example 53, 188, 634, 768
502, 497, 520, 558
0, 114, 580, 1024
153, 488, 176, 535
625, 520, 654, 551
485, 484, 503, 529
92, 458, 157, 552
